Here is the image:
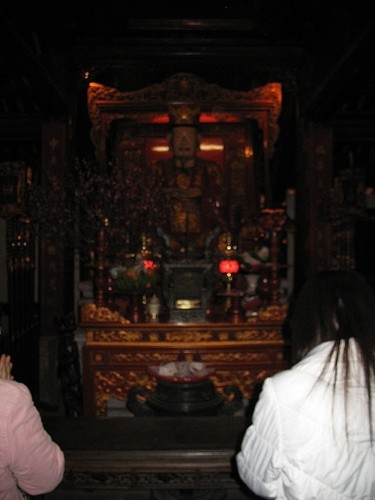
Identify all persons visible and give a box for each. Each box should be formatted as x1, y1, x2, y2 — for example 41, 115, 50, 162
0, 353, 66, 500
235, 272, 375, 500
137, 103, 229, 309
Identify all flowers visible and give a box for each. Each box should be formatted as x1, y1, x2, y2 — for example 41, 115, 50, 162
109, 259, 162, 291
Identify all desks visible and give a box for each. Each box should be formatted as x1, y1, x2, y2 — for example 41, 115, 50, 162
45, 416, 246, 491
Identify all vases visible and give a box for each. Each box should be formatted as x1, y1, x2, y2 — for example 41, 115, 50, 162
126, 293, 145, 323
